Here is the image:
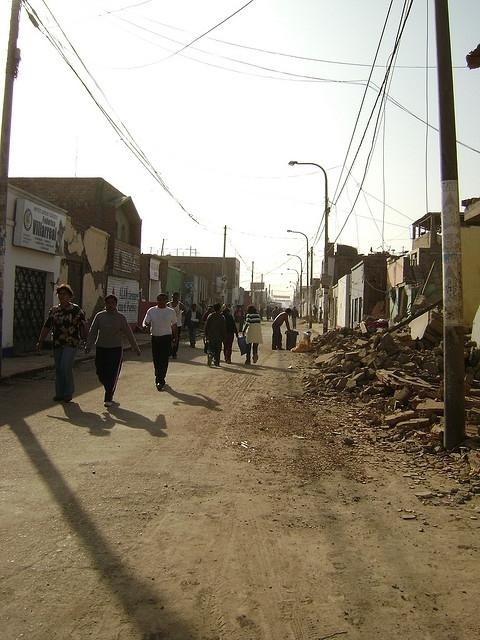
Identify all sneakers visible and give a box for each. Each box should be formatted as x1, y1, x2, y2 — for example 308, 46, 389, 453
103, 400, 120, 407
208, 357, 232, 367
52, 393, 73, 404
155, 378, 166, 390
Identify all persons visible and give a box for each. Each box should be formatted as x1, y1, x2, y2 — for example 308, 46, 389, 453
184, 303, 202, 348
320, 306, 323, 320
142, 292, 178, 390
260, 306, 280, 321
222, 315, 238, 364
167, 291, 185, 359
203, 303, 226, 367
242, 304, 264, 364
84, 294, 142, 407
34, 282, 88, 404
272, 308, 292, 350
290, 306, 299, 329
202, 303, 246, 332
312, 304, 318, 320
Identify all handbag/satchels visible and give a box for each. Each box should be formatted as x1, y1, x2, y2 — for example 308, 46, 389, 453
238, 334, 249, 355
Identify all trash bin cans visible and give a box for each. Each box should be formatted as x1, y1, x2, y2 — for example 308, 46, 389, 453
285, 330, 299, 350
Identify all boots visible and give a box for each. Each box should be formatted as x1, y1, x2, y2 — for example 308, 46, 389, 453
244, 352, 259, 364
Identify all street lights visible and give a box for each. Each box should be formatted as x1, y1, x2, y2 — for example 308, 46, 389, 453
288, 161, 329, 334
